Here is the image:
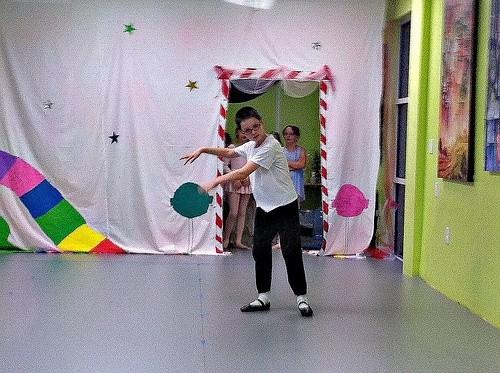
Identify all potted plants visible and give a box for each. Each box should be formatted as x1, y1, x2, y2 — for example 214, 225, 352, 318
309, 151, 322, 184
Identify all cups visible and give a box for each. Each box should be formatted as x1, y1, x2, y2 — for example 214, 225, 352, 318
311, 172, 316, 177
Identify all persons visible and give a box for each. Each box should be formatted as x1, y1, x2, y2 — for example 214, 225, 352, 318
180, 107, 314, 317
279, 126, 307, 203
223, 126, 252, 249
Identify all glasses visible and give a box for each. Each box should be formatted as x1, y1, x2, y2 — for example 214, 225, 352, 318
240, 123, 260, 134
283, 132, 294, 135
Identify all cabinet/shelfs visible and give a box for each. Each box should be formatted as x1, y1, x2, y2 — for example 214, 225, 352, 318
298, 184, 322, 229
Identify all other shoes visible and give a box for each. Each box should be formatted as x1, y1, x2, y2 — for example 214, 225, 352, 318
297, 301, 313, 317
240, 298, 271, 312
